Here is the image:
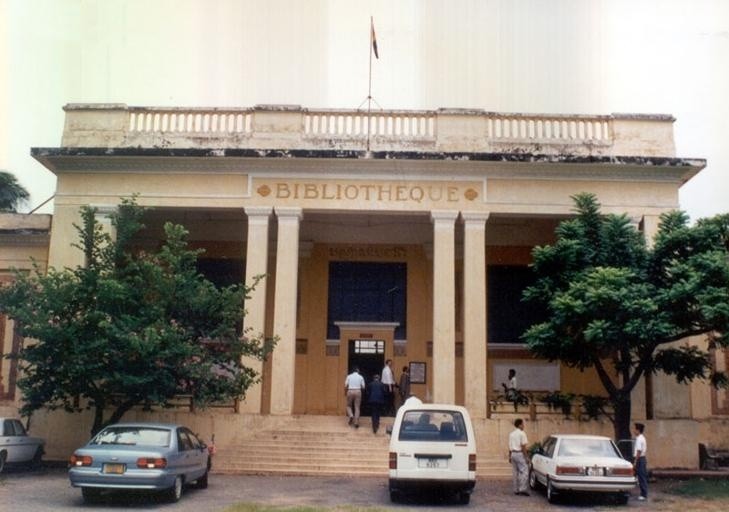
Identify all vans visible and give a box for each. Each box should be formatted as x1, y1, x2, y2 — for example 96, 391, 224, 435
382, 402, 477, 508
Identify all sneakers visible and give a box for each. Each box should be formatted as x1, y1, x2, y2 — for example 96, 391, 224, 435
637, 495, 647, 500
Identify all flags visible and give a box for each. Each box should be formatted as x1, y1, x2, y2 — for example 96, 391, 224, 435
373, 28, 378, 59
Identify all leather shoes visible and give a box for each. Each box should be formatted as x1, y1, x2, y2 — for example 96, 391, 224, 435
519, 491, 530, 496
514, 491, 519, 495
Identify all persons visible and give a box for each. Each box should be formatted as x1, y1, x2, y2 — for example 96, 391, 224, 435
633, 423, 648, 500
501, 369, 518, 401
398, 366, 411, 404
365, 374, 386, 432
344, 367, 365, 428
380, 359, 396, 416
508, 418, 530, 496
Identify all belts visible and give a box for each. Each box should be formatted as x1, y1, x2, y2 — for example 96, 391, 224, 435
511, 449, 523, 453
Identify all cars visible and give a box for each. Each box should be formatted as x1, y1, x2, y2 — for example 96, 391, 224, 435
0, 416, 47, 472
62, 419, 212, 504
525, 431, 638, 507
